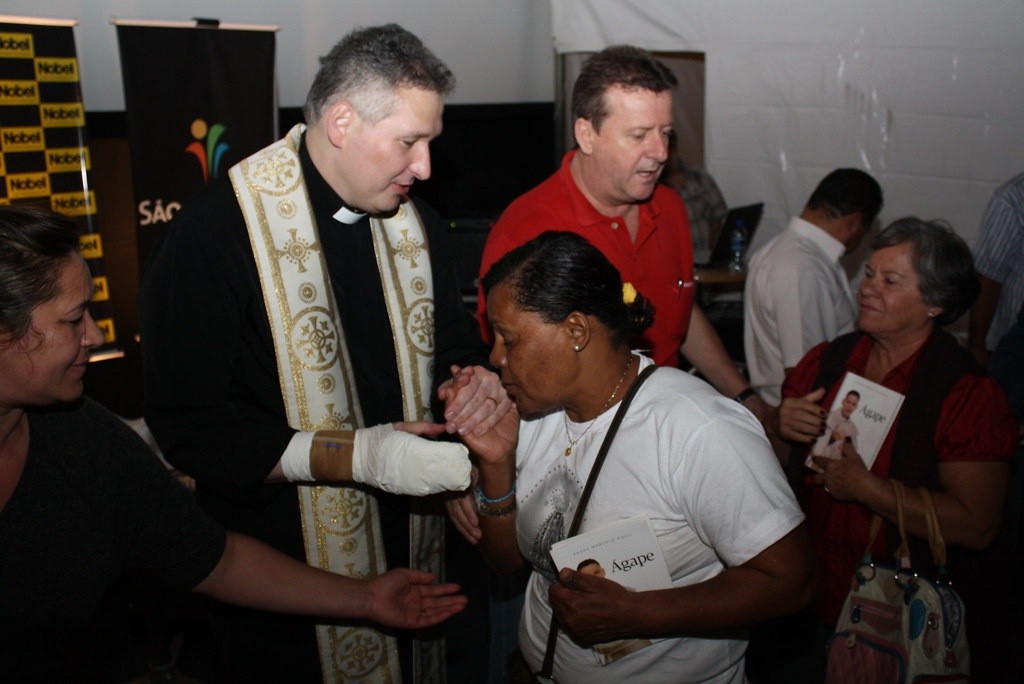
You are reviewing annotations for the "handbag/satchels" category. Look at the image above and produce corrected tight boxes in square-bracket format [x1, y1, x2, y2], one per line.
[828, 479, 971, 684]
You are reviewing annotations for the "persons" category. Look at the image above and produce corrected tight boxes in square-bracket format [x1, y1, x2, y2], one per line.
[139, 24, 513, 684]
[575, 559, 654, 665]
[0, 203, 471, 684]
[475, 44, 793, 464]
[777, 212, 1023, 684]
[447, 229, 811, 683]
[805, 390, 861, 474]
[740, 167, 884, 405]
[658, 127, 729, 267]
[967, 171, 1024, 684]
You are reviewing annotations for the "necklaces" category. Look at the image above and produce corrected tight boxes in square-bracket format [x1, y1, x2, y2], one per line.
[564, 356, 633, 456]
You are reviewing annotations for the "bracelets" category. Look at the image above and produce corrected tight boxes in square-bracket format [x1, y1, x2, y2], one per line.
[473, 485, 516, 519]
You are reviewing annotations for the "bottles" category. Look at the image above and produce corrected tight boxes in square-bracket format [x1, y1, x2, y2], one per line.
[730, 228, 745, 276]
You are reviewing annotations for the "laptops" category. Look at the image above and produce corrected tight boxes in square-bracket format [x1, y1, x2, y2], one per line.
[691, 203, 766, 270]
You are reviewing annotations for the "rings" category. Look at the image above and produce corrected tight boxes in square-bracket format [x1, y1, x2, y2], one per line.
[487, 396, 498, 407]
[823, 484, 830, 492]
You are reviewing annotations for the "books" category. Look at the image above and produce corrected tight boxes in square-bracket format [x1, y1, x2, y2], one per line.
[550, 515, 676, 665]
[804, 370, 905, 474]
